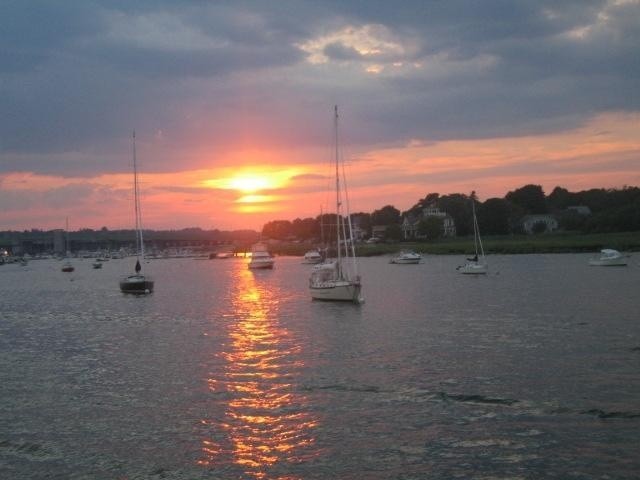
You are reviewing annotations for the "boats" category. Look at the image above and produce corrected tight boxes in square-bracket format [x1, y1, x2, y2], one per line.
[82, 248, 121, 262]
[247, 244, 273, 270]
[390, 250, 421, 264]
[62, 265, 74, 272]
[92, 263, 102, 268]
[300, 251, 323, 264]
[589, 247, 633, 267]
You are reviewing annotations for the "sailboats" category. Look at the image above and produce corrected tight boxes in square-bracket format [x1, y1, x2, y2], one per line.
[120, 128, 154, 294]
[455, 197, 490, 274]
[307, 104, 365, 304]
